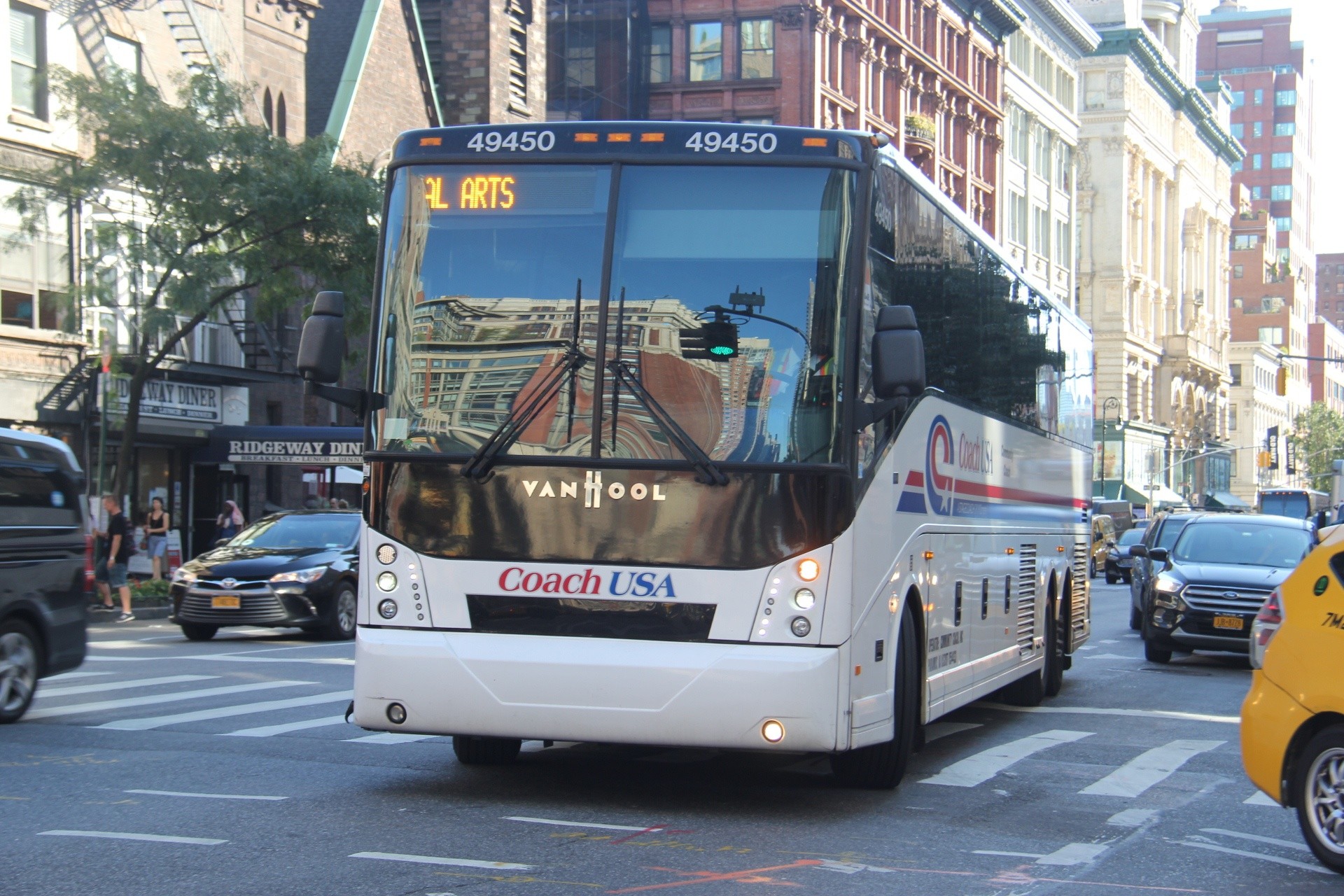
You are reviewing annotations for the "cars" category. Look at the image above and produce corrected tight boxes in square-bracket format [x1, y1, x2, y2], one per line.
[1240, 524, 1344, 874]
[1141, 512, 1319, 668]
[1089, 514, 1151, 584]
[167, 508, 362, 641]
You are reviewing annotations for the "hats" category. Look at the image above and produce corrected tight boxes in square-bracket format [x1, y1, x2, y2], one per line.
[340, 499, 348, 508]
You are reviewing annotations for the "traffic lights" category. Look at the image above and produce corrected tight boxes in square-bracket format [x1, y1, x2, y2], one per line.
[680, 322, 739, 362]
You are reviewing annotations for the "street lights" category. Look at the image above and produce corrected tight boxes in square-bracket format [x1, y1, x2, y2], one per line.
[1187, 427, 1207, 500]
[1102, 397, 1123, 495]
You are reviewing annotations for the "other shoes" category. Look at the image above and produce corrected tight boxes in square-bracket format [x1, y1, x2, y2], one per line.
[94, 603, 113, 609]
[113, 614, 136, 623]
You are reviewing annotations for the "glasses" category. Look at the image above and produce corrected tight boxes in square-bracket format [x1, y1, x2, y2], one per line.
[331, 503, 335, 504]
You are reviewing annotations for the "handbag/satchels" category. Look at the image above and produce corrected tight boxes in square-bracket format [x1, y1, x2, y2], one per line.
[208, 537, 219, 548]
[121, 521, 139, 557]
[140, 538, 147, 551]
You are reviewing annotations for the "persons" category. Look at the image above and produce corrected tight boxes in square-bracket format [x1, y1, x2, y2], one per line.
[142, 496, 170, 580]
[92, 492, 135, 623]
[215, 501, 244, 546]
[306, 498, 349, 509]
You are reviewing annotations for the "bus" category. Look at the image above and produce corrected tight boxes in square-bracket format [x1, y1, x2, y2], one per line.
[1256, 490, 1330, 520]
[298, 126, 1093, 790]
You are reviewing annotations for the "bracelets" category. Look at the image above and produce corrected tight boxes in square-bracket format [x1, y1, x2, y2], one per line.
[110, 557, 115, 560]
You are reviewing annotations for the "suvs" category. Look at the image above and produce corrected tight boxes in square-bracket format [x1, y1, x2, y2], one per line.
[0, 427, 95, 723]
[1129, 506, 1246, 643]
